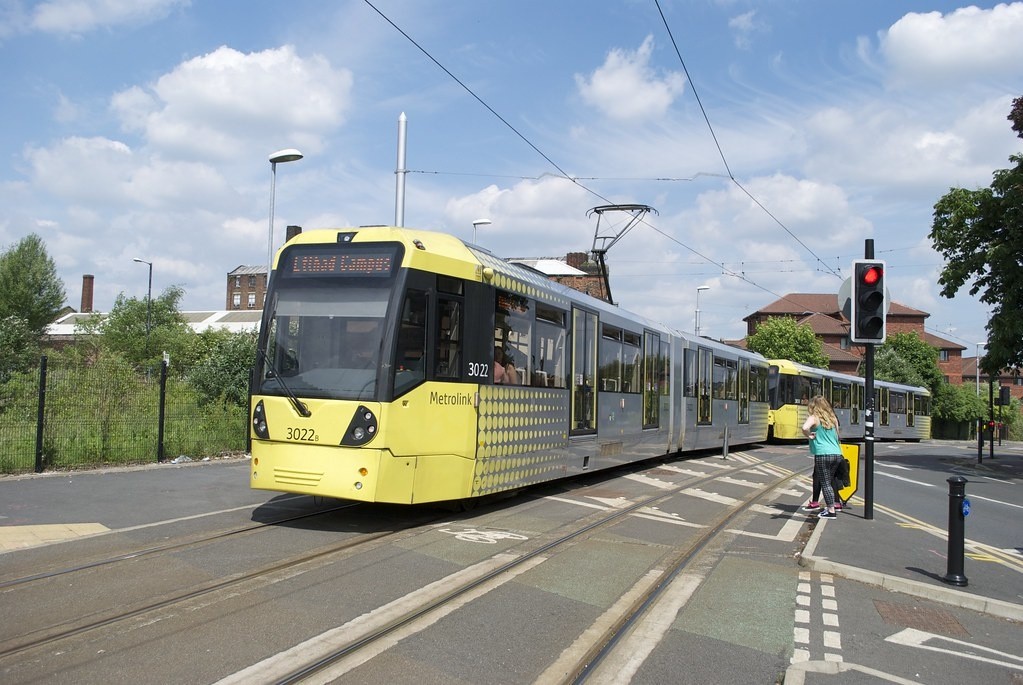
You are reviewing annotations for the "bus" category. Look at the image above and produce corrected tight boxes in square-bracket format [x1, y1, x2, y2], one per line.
[765, 323, 931, 444]
[247, 205, 770, 505]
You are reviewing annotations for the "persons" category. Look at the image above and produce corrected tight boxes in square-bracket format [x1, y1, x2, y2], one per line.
[494, 346, 517, 385]
[801, 395, 842, 520]
[801, 393, 808, 405]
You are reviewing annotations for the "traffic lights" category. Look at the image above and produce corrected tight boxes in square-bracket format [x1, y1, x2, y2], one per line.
[854, 262, 884, 341]
[987, 420, 995, 432]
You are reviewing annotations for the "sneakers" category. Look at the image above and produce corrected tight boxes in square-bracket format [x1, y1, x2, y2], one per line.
[817, 509, 837, 519]
[834, 504, 842, 511]
[801, 500, 821, 511]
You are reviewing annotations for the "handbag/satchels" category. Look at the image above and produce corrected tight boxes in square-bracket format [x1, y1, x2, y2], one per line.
[839, 457, 852, 487]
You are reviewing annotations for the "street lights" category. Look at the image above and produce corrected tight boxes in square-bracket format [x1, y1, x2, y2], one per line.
[132, 257, 153, 375]
[694, 285, 711, 335]
[261, 147, 304, 379]
[975, 342, 989, 444]
[473, 218, 492, 246]
